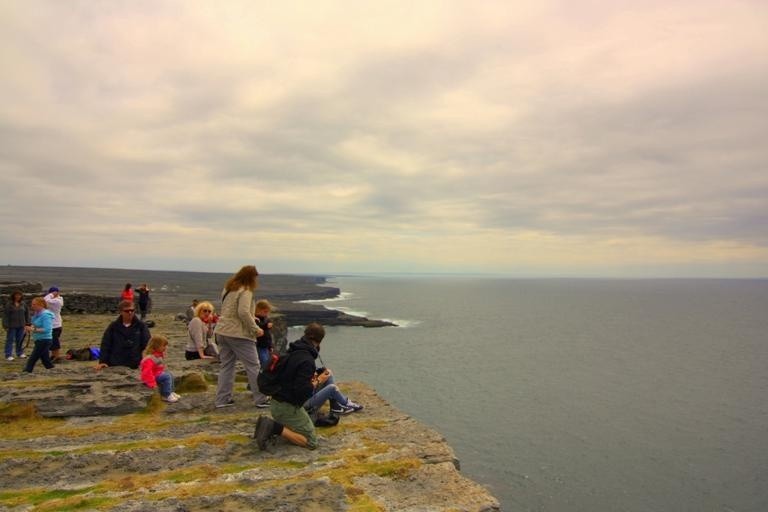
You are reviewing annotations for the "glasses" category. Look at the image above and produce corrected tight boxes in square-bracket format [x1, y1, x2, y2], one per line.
[124, 310, 134, 313]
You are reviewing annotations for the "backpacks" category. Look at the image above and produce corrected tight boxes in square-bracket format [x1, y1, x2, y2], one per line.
[257, 352, 290, 396]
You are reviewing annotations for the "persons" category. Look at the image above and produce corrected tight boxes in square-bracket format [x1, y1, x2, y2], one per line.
[252, 320, 327, 446]
[215, 265, 272, 408]
[304, 366, 361, 414]
[0, 283, 276, 402]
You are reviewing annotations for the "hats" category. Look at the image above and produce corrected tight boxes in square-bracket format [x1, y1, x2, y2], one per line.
[49, 288, 58, 291]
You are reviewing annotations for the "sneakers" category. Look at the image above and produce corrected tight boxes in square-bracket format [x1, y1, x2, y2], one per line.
[20, 354, 27, 358]
[254, 415, 274, 450]
[161, 392, 181, 403]
[5, 356, 14, 361]
[255, 398, 272, 408]
[216, 400, 235, 407]
[330, 397, 363, 415]
